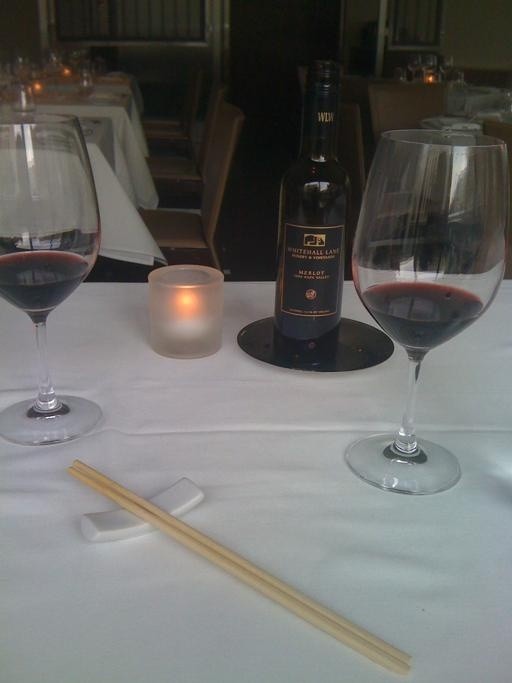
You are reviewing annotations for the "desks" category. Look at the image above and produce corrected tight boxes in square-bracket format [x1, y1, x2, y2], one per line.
[431, 79, 511, 144]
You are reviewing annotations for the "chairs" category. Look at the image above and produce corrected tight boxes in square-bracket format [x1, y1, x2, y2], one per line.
[297, 62, 368, 279]
[140, 61, 245, 272]
[367, 76, 448, 155]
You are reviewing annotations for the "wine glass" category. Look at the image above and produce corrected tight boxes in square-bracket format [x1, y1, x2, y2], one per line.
[0, 112, 114, 454]
[1, 47, 101, 116]
[344, 128, 509, 495]
[398, 49, 508, 124]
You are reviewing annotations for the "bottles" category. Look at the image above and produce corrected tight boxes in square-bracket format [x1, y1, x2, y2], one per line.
[274, 29, 353, 342]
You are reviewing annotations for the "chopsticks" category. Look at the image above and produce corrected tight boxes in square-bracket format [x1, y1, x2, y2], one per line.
[64, 458, 419, 678]
